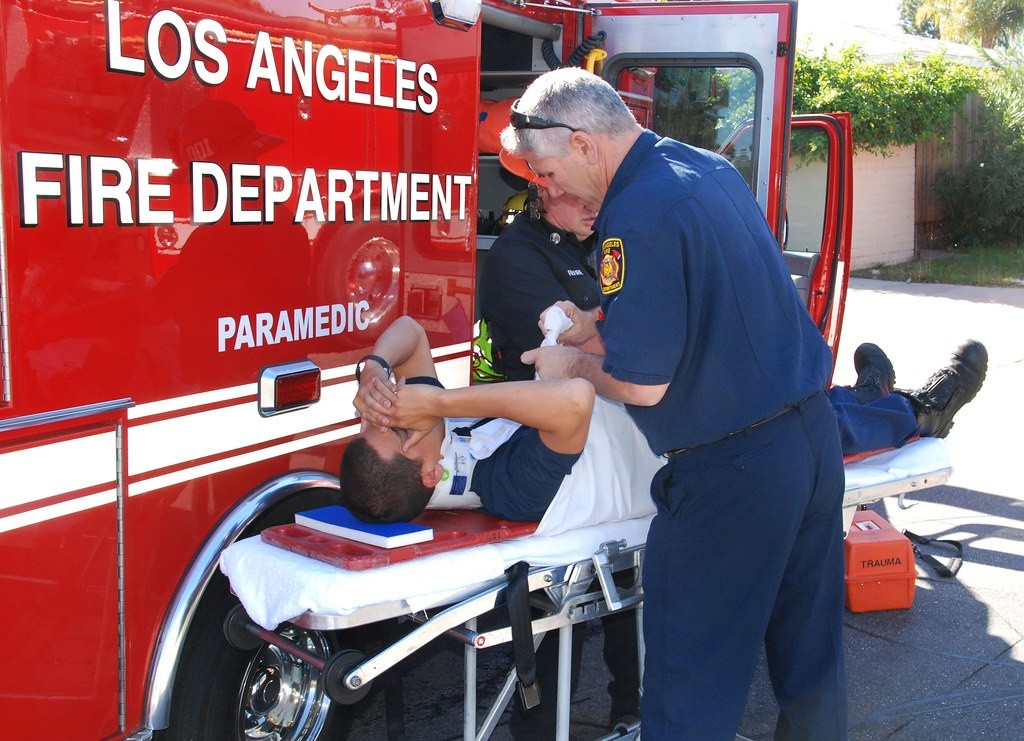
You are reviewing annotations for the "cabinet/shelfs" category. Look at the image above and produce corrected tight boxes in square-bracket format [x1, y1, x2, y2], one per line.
[477, 69, 547, 249]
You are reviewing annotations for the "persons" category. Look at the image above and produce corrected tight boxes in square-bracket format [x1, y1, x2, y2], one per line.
[503, 67, 847, 740]
[480, 177, 642, 741]
[338, 315, 988, 526]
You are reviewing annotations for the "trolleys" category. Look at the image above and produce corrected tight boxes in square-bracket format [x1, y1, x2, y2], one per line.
[217, 436, 958, 741]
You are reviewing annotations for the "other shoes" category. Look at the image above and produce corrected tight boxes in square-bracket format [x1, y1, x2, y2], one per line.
[895, 339, 988, 438]
[854, 342, 896, 396]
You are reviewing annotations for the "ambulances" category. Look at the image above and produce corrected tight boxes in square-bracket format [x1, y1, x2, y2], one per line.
[0, 0, 853, 739]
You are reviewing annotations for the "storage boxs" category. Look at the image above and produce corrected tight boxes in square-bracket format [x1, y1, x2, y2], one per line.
[845, 510, 920, 611]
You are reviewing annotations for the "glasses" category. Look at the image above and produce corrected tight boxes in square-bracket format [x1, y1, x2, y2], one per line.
[509, 98, 577, 132]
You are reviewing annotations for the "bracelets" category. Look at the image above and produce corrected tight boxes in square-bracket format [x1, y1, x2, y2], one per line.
[355, 355, 392, 385]
